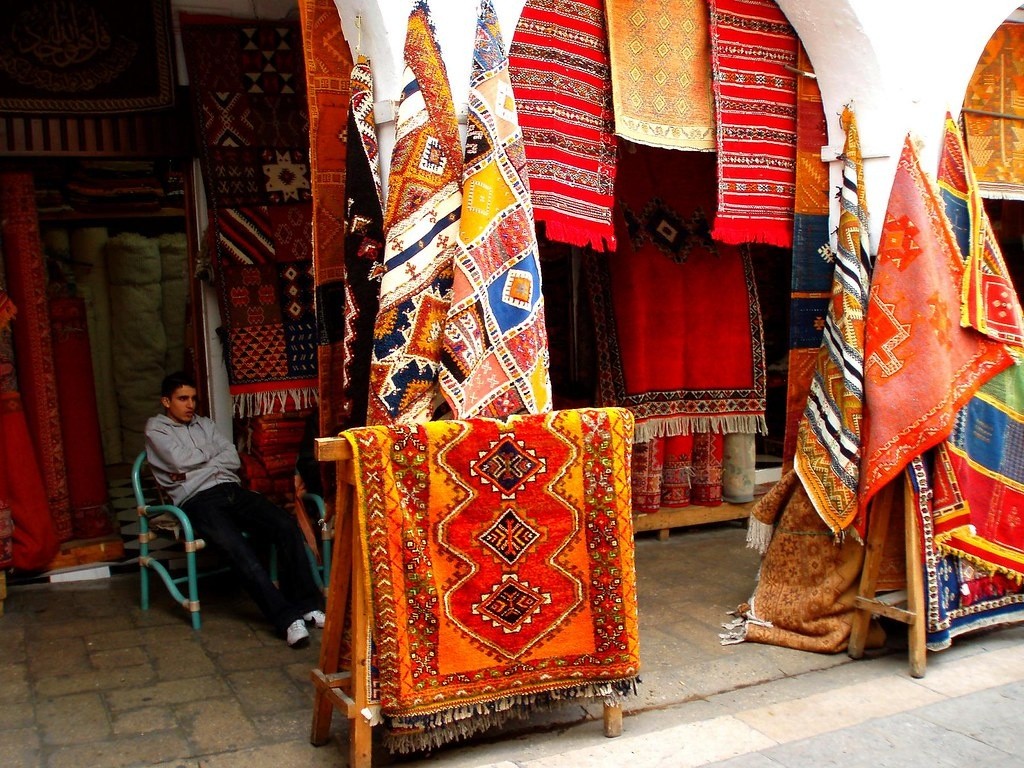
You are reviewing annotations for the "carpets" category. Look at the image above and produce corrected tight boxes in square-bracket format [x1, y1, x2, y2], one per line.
[1, 0, 1023, 755]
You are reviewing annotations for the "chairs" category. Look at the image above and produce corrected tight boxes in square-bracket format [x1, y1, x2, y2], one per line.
[293, 451, 331, 608]
[132, 449, 279, 630]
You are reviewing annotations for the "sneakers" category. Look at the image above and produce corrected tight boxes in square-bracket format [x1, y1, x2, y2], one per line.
[286, 619, 309, 646]
[303, 609, 325, 628]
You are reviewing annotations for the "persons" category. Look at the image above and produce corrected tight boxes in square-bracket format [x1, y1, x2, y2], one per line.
[144, 372, 327, 647]
